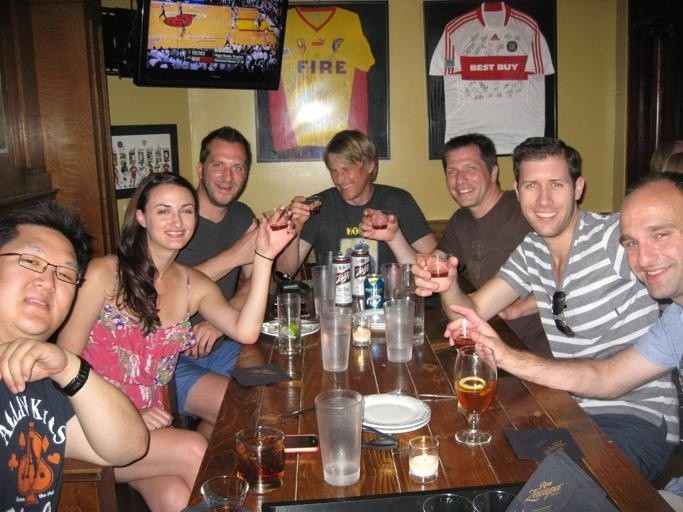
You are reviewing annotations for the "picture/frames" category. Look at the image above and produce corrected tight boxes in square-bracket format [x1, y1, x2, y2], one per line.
[107, 122, 180, 199]
[252, 1, 392, 164]
[422, 1, 558, 160]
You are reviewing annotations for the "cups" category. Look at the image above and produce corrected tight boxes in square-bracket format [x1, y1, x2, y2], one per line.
[314, 389, 363, 486]
[368, 209, 389, 229]
[304, 195, 321, 215]
[473, 491, 525, 512]
[199, 474, 249, 512]
[273, 242, 452, 370]
[406, 434, 439, 486]
[262, 208, 289, 231]
[421, 493, 476, 512]
[235, 426, 285, 494]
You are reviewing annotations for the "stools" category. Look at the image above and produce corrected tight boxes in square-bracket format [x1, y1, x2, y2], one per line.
[51, 450, 121, 512]
[161, 369, 181, 430]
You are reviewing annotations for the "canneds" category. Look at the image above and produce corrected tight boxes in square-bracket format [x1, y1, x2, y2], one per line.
[351, 250, 371, 298]
[332, 256, 353, 307]
[364, 274, 385, 310]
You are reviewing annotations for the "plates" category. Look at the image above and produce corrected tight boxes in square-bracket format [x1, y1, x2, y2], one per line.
[359, 393, 432, 435]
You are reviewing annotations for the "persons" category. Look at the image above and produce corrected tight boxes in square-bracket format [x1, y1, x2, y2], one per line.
[114, 138, 170, 188]
[411, 137, 683, 484]
[358, 133, 556, 362]
[147, 0, 282, 75]
[273, 129, 438, 283]
[57, 172, 298, 511]
[0, 196, 151, 512]
[167, 127, 260, 440]
[445, 169, 683, 511]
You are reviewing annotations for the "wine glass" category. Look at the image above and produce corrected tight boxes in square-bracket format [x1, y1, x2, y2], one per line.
[452, 345, 495, 446]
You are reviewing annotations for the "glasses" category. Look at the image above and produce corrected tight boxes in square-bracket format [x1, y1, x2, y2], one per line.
[0, 248, 85, 287]
[552, 290, 578, 339]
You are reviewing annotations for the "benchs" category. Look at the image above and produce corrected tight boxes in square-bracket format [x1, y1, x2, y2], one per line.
[267, 217, 454, 294]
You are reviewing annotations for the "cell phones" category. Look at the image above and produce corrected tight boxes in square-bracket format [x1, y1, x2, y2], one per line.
[269, 309, 312, 320]
[283, 434, 320, 453]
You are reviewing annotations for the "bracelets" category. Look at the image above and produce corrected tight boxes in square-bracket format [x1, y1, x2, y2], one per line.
[53, 355, 90, 397]
[255, 251, 273, 261]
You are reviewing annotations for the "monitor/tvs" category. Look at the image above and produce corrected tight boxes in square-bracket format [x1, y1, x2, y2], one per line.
[133, 0, 288, 90]
